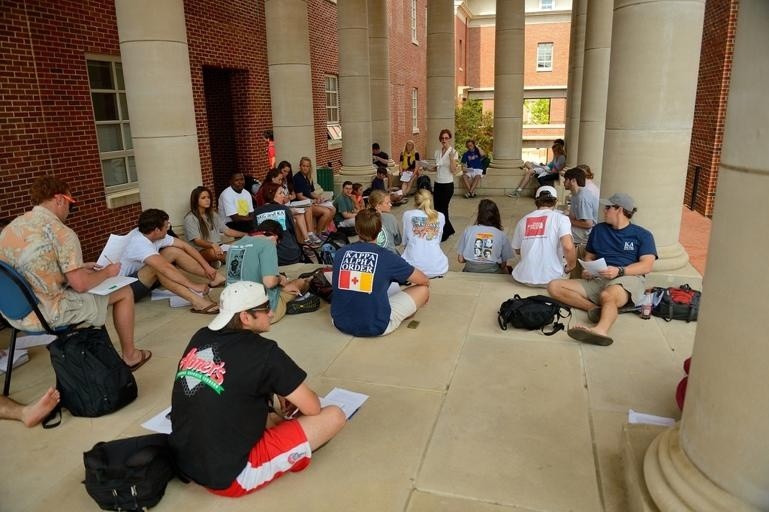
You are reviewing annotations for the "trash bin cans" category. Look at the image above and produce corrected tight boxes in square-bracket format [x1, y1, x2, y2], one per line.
[317, 166, 334, 191]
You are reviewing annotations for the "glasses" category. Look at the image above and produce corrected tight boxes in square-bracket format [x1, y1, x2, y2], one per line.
[248, 304, 272, 314]
[53, 193, 81, 213]
[440, 137, 450, 140]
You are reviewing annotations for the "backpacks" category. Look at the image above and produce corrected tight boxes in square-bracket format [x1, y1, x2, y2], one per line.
[495, 291, 572, 337]
[320, 230, 350, 266]
[296, 266, 334, 304]
[42, 325, 140, 418]
[81, 432, 190, 512]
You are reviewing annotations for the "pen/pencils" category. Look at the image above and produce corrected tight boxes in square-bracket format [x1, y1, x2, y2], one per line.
[287, 278, 303, 297]
[104, 255, 113, 264]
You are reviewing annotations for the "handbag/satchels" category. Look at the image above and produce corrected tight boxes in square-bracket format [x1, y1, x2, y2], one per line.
[651, 283, 701, 323]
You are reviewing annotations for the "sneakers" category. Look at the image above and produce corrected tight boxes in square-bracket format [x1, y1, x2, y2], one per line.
[307, 231, 322, 244]
[285, 291, 321, 314]
[464, 192, 472, 198]
[472, 192, 475, 198]
[304, 239, 320, 249]
[507, 191, 521, 198]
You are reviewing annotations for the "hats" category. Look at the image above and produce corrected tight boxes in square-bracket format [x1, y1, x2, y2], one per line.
[534, 185, 558, 199]
[248, 219, 284, 239]
[206, 279, 270, 332]
[597, 191, 635, 214]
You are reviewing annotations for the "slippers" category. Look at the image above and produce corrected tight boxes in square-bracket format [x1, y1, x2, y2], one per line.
[123, 348, 153, 372]
[587, 306, 602, 323]
[566, 326, 615, 348]
[189, 302, 220, 314]
[207, 279, 226, 288]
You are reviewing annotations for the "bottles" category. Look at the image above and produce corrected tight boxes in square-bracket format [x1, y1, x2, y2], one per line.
[640, 287, 652, 320]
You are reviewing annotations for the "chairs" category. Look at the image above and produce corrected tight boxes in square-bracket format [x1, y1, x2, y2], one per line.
[0, 259, 95, 401]
